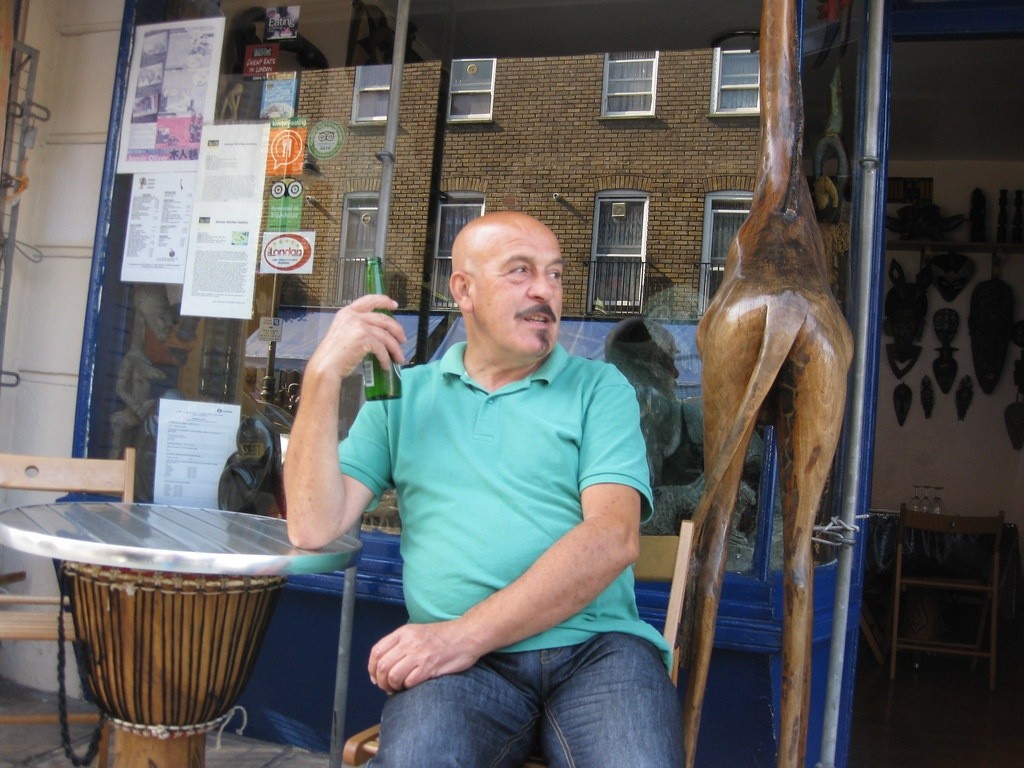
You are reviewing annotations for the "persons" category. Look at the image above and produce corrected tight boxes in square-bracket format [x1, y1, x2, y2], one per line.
[283, 210, 688, 768]
[600, 316, 763, 536]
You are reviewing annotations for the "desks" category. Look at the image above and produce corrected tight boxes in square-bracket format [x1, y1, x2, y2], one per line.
[0, 499, 364, 768]
[866, 511, 1018, 658]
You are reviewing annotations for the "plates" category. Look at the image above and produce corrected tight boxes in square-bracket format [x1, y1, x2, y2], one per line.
[870, 508, 900, 513]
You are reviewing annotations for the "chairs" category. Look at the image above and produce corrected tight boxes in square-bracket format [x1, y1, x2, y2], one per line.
[1, 445, 137, 768]
[891, 502, 1005, 690]
[342, 518, 696, 767]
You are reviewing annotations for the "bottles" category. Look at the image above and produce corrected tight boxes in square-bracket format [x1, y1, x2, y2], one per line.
[362, 257, 402, 401]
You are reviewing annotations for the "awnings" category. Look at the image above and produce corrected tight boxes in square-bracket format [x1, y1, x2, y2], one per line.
[241, 306, 701, 399]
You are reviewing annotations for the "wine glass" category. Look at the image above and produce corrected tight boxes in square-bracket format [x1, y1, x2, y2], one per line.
[931, 487, 945, 515]
[909, 486, 922, 512]
[920, 486, 931, 514]
